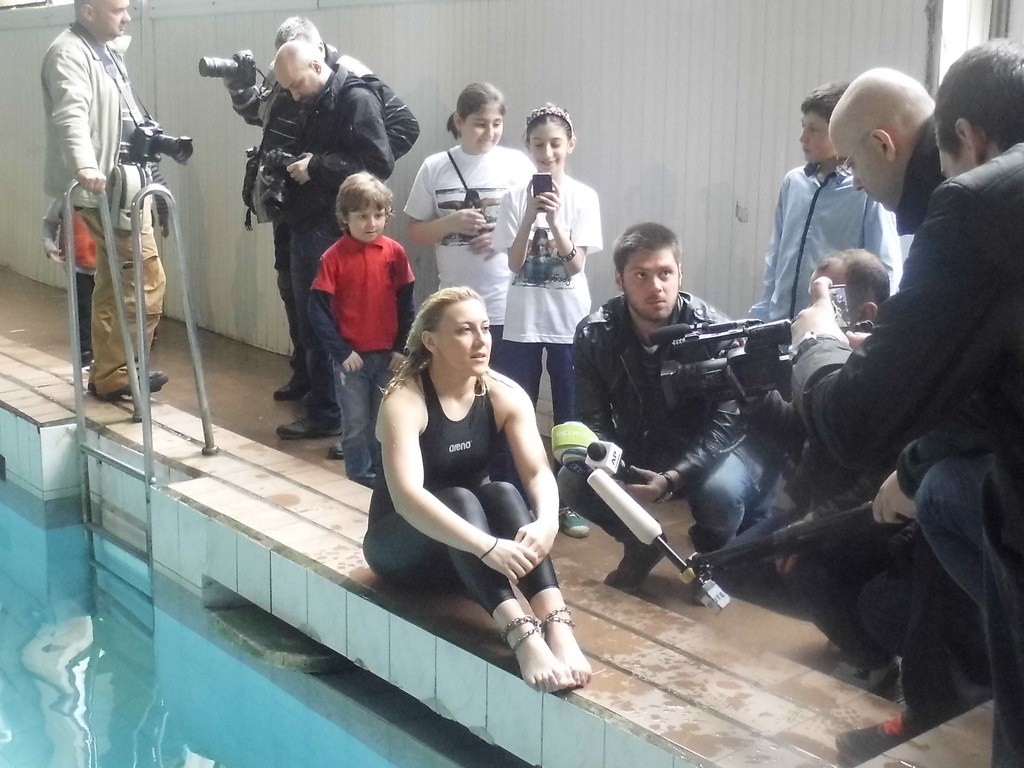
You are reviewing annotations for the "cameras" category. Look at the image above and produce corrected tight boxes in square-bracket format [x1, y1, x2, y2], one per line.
[130, 120, 193, 165]
[265, 149, 302, 203]
[199, 50, 256, 89]
[827, 283, 852, 334]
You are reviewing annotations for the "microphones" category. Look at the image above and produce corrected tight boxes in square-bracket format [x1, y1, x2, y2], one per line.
[586, 468, 696, 584]
[648, 322, 708, 346]
[584, 441, 647, 487]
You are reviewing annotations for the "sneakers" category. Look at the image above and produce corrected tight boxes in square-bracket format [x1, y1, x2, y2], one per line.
[277, 416, 342, 439]
[828, 640, 900, 696]
[835, 707, 948, 765]
[559, 506, 590, 539]
[328, 440, 344, 459]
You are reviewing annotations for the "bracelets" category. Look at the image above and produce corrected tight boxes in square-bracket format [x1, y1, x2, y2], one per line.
[480, 538, 498, 560]
[556, 243, 577, 261]
[786, 332, 840, 367]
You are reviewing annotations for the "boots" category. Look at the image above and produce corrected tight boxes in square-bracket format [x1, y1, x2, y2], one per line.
[604, 519, 669, 593]
[679, 526, 736, 602]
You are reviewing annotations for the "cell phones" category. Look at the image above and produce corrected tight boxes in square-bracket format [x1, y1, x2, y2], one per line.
[532, 173, 553, 199]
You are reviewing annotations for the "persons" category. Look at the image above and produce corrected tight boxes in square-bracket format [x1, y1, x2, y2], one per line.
[363, 285, 591, 694]
[402, 80, 539, 374]
[42, 0, 171, 401]
[493, 103, 604, 471]
[556, 222, 772, 605]
[224, 17, 419, 487]
[747, 40, 1024, 767]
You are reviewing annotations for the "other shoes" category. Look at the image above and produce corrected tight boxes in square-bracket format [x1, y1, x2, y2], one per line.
[81, 351, 92, 364]
[88, 372, 169, 400]
[273, 377, 312, 408]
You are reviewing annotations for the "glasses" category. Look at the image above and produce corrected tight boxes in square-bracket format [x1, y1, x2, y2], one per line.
[834, 130, 873, 177]
